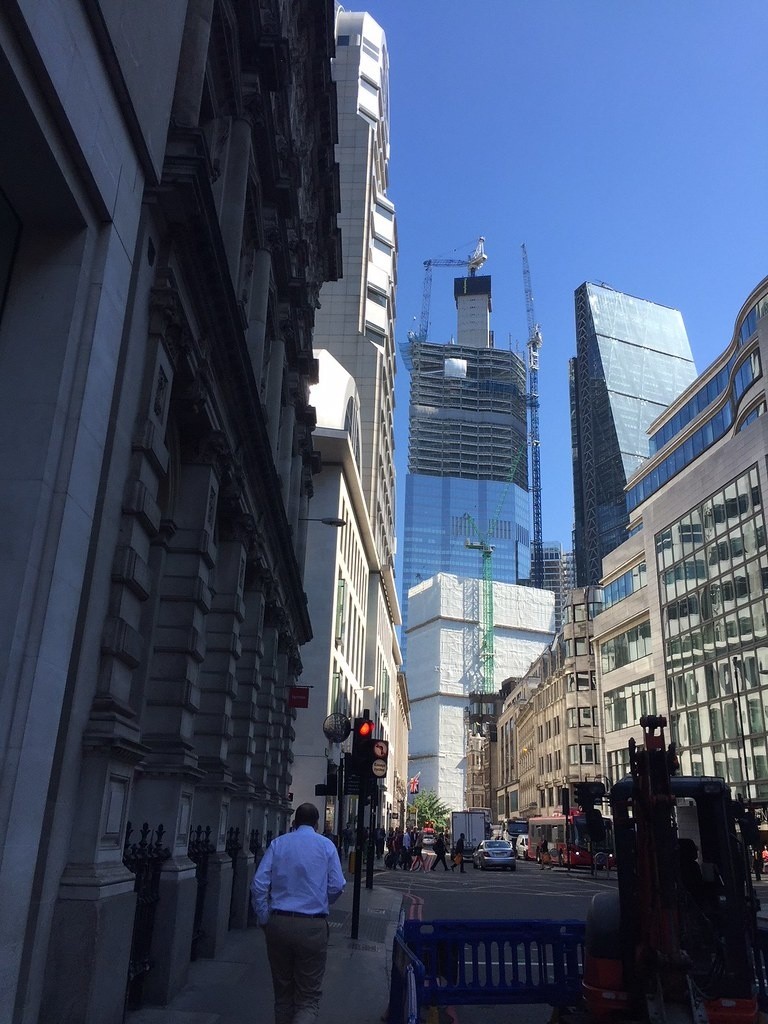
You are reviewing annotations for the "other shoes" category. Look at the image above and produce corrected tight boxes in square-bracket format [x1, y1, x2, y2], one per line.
[431, 867, 436, 871]
[539, 868, 544, 870]
[461, 870, 467, 873]
[445, 868, 450, 871]
[451, 866, 454, 871]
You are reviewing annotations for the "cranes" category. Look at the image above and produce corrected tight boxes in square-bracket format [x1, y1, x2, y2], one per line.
[459, 439, 534, 690]
[519, 242, 545, 587]
[418, 236, 487, 344]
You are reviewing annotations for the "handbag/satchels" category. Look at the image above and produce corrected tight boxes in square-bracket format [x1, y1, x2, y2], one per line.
[414, 847, 420, 855]
[433, 844, 441, 854]
[454, 855, 462, 865]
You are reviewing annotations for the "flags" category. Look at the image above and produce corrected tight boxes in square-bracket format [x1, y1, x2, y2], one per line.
[410, 775, 419, 794]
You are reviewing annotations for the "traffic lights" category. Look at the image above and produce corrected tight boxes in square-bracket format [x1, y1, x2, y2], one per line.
[573, 783, 583, 805]
[351, 719, 374, 772]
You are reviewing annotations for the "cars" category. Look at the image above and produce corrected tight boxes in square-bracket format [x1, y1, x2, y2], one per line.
[422, 835, 436, 845]
[472, 840, 516, 871]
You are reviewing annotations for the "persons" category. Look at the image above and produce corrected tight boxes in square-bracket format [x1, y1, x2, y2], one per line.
[430, 833, 451, 871]
[250, 803, 348, 1024]
[539, 835, 553, 870]
[388, 825, 428, 874]
[323, 823, 385, 863]
[450, 833, 467, 873]
[753, 858, 763, 881]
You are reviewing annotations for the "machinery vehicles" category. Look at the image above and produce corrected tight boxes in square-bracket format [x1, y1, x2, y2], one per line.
[551, 715, 768, 1024]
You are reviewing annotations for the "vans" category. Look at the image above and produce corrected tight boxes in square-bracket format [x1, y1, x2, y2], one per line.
[516, 834, 529, 860]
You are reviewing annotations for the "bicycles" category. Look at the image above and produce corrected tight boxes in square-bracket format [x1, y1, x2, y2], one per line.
[384, 849, 420, 871]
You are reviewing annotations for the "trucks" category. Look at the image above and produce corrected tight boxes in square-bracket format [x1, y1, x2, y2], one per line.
[492, 825, 503, 840]
[497, 817, 528, 850]
[451, 811, 486, 861]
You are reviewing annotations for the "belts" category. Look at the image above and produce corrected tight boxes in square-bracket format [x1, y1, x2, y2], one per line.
[271, 910, 327, 918]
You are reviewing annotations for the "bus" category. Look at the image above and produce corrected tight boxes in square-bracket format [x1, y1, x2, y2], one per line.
[528, 816, 615, 870]
[467, 807, 492, 840]
